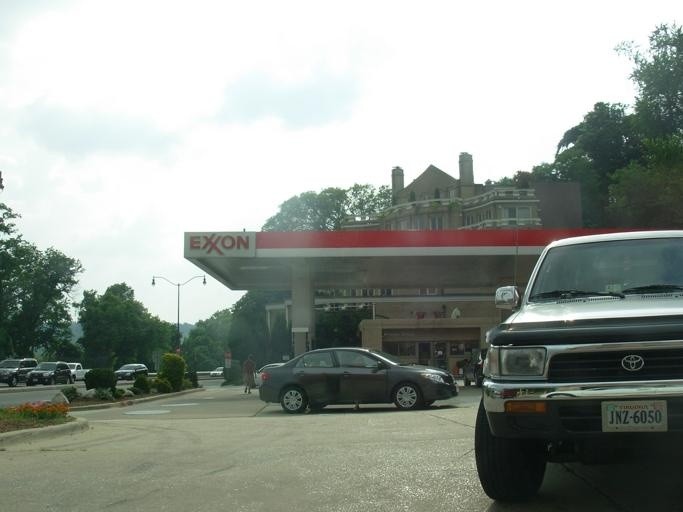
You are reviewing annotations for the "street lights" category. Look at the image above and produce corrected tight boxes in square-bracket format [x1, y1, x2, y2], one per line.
[151, 274, 206, 355]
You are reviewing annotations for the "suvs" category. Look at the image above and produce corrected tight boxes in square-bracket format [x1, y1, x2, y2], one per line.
[0, 358, 38, 387]
[24, 361, 71, 386]
[474, 229, 682, 503]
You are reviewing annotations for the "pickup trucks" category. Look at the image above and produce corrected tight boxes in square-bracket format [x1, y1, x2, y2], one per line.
[66, 362, 92, 384]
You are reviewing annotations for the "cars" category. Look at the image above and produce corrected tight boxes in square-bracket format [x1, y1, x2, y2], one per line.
[251, 362, 285, 388]
[462, 348, 487, 387]
[209, 366, 224, 377]
[113, 363, 148, 380]
[259, 347, 459, 414]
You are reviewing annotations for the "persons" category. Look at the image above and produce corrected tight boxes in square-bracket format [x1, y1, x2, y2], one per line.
[241, 353, 256, 394]
